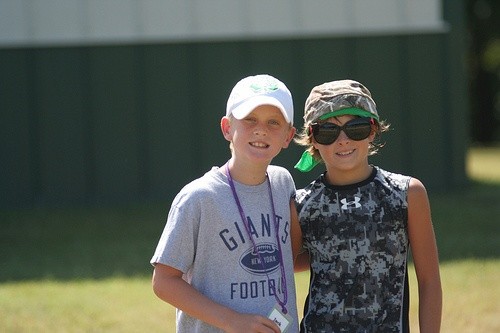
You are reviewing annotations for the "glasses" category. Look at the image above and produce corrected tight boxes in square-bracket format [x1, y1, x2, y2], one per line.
[310, 117, 371, 145]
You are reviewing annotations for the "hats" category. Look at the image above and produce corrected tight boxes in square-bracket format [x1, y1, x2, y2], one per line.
[226, 74, 294, 127]
[294, 80, 379, 173]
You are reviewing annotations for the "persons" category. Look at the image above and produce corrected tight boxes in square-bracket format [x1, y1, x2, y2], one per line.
[293, 80, 443, 332]
[148, 75, 308, 332]
